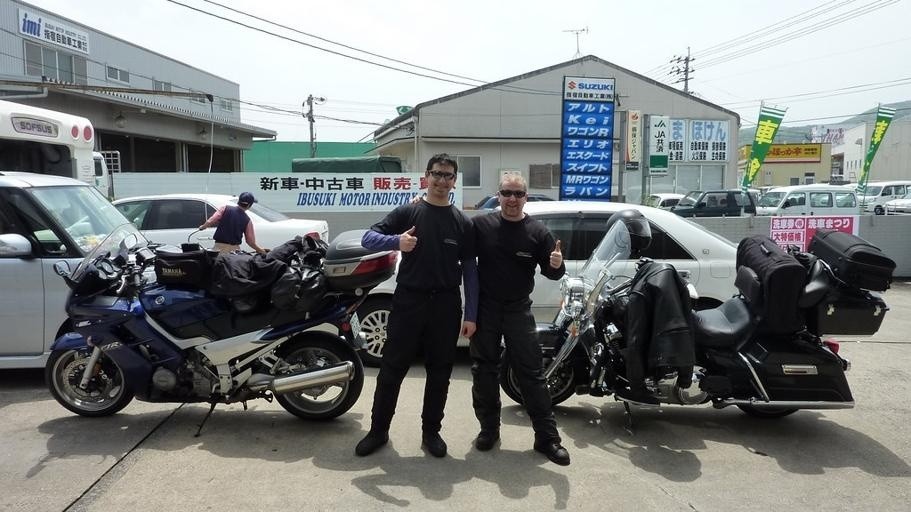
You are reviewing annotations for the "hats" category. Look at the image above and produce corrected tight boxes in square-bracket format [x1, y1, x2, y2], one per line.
[238, 192, 257, 207]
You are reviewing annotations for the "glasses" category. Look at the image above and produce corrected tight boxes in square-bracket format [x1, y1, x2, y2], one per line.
[430, 170, 456, 180]
[499, 190, 526, 198]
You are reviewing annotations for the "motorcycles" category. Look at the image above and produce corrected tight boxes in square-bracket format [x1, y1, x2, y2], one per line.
[493, 219, 895, 440]
[43, 221, 396, 438]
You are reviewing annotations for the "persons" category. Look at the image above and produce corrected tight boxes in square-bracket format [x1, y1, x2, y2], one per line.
[354, 152, 480, 462]
[194, 188, 266, 256]
[408, 193, 423, 204]
[465, 170, 571, 467]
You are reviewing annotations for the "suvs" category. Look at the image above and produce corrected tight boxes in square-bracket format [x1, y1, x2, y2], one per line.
[660, 189, 756, 216]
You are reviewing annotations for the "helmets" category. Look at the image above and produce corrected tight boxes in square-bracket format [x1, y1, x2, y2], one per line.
[605, 209, 652, 252]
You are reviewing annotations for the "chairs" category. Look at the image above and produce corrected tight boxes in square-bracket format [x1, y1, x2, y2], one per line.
[793, 250, 830, 310]
[692, 263, 764, 344]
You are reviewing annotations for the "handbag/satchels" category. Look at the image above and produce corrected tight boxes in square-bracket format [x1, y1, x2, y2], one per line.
[735, 234, 804, 334]
[153, 245, 212, 290]
[271, 251, 327, 312]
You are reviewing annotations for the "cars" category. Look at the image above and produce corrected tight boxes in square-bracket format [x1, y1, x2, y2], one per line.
[62, 195, 327, 256]
[351, 199, 748, 368]
[648, 193, 695, 210]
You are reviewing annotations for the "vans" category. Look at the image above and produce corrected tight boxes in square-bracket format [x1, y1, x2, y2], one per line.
[750, 180, 911, 215]
[0, 170, 184, 369]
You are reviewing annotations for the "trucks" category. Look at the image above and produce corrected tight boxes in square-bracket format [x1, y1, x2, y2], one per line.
[0, 99, 112, 202]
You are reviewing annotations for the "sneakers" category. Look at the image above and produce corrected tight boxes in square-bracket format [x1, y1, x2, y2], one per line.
[356, 428, 388, 457]
[422, 433, 446, 456]
[477, 430, 499, 450]
[534, 440, 570, 465]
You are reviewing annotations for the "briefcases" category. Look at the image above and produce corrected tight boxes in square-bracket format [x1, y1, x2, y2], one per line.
[808, 228, 895, 291]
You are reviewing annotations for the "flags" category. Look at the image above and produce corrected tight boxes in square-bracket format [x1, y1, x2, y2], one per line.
[856, 106, 898, 193]
[741, 104, 785, 186]
